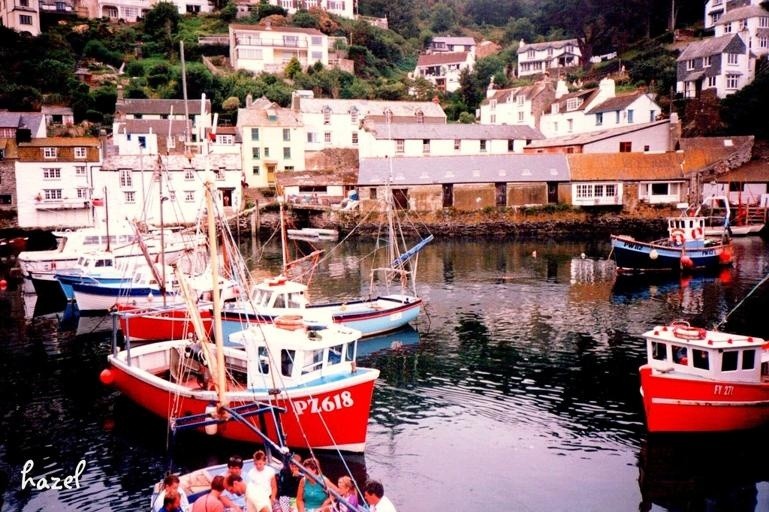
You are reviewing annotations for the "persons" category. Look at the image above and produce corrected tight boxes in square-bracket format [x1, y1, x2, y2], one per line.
[152, 447, 399, 512]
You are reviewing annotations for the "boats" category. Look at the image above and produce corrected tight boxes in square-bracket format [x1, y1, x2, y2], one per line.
[639, 325, 769, 433]
[610, 195, 736, 277]
